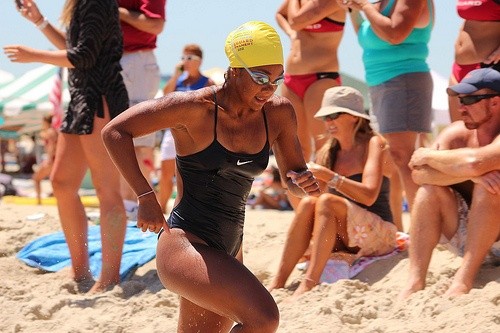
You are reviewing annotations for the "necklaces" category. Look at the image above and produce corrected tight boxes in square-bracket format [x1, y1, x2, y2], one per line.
[349, 0, 386, 13]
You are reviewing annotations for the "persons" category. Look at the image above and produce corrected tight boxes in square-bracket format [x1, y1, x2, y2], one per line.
[400, 67, 500, 300]
[101, 20, 320, 333]
[0, 135, 39, 176]
[93, 0, 166, 226]
[32, 115, 59, 205]
[337, 0, 435, 232]
[449, 0, 500, 122]
[275, 0, 349, 165]
[266, 87, 398, 298]
[250, 165, 294, 211]
[4, 0, 130, 296]
[156, 43, 216, 214]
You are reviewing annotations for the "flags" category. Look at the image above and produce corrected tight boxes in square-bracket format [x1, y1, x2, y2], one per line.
[48, 66, 64, 129]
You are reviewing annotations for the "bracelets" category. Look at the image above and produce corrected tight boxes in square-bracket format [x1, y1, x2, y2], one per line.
[136, 190, 155, 199]
[36, 18, 49, 31]
[34, 15, 42, 23]
[336, 176, 345, 191]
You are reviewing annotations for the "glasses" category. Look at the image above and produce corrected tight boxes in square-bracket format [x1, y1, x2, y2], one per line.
[181, 56, 198, 60]
[459, 94, 500, 105]
[231, 37, 284, 86]
[322, 112, 348, 120]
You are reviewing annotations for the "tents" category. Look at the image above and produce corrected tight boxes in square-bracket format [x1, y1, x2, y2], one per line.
[0, 63, 175, 139]
[200, 66, 228, 86]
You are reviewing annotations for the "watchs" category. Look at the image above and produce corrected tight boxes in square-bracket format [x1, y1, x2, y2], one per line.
[327, 173, 339, 188]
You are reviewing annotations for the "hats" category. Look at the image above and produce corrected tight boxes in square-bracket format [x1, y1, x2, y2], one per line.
[313, 86, 371, 120]
[446, 67, 500, 96]
[225, 21, 284, 68]
